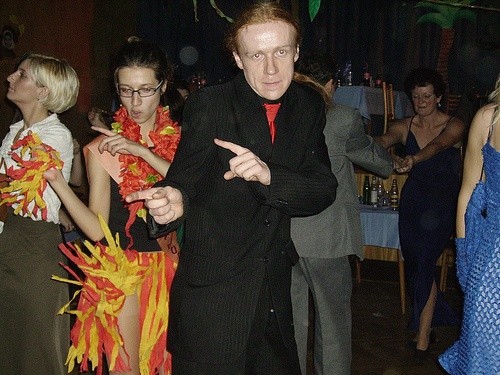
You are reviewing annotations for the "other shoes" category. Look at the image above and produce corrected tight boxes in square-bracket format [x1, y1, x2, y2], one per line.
[409, 326, 435, 360]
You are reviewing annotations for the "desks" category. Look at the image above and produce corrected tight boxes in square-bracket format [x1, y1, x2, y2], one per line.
[354, 171, 409, 315]
[333, 85, 416, 135]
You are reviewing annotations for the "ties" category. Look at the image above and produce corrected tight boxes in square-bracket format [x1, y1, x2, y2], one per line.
[263, 102, 282, 145]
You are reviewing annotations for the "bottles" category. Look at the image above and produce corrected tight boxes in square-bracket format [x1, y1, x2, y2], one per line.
[363, 176, 371, 205]
[376, 75, 381, 88]
[363, 64, 371, 86]
[371, 176, 378, 205]
[377, 177, 384, 206]
[391, 179, 399, 210]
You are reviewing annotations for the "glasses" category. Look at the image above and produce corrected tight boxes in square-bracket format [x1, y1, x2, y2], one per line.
[116, 79, 163, 98]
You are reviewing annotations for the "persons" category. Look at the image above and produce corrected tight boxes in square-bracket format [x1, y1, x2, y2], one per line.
[0, 42, 500, 375]
[124, 3, 338, 375]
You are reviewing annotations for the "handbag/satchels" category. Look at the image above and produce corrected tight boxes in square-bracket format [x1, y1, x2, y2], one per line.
[0, 157, 11, 223]
